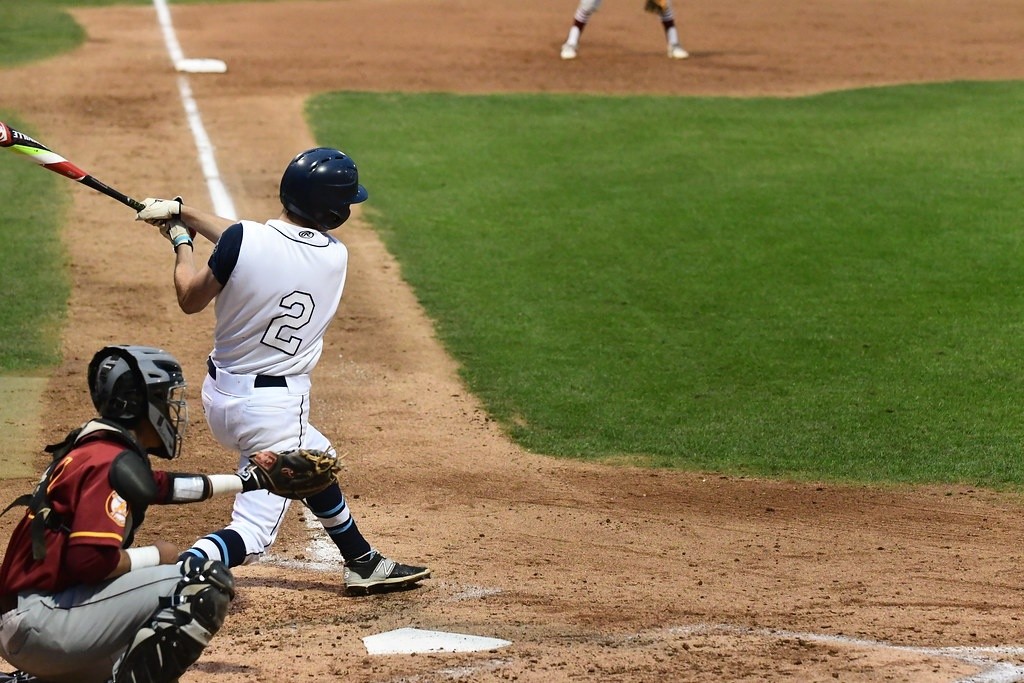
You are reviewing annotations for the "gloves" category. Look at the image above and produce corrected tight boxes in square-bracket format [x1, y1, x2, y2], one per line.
[135, 197, 182, 228]
[159, 196, 197, 253]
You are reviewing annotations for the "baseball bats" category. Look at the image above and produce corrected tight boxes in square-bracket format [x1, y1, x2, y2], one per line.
[0, 121, 145, 211]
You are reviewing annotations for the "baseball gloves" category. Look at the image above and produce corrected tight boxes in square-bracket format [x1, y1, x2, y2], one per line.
[249, 449, 342, 500]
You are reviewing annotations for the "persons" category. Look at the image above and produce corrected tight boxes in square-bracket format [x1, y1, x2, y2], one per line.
[135, 147, 431, 594]
[560, 0, 689, 59]
[0, 346, 345, 683]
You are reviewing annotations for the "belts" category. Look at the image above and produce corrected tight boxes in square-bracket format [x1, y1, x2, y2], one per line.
[0, 595, 18, 614]
[208, 356, 287, 387]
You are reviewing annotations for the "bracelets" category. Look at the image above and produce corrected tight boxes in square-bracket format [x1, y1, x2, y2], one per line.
[171, 234, 194, 253]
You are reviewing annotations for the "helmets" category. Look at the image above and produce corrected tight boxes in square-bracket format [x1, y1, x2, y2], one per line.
[280, 148, 368, 229]
[88, 345, 187, 460]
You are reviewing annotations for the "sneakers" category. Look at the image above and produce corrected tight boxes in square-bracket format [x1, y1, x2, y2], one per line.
[344, 551, 430, 596]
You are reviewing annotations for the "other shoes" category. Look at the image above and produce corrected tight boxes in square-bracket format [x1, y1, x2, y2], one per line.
[667, 45, 689, 58]
[561, 42, 577, 59]
